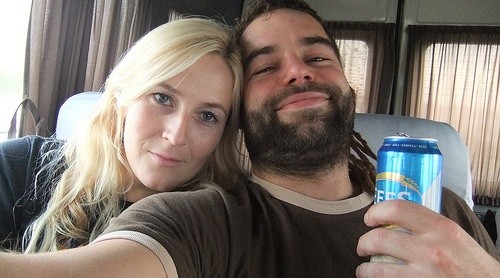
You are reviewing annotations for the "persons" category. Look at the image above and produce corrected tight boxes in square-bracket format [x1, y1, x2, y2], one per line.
[0, 1, 500, 278]
[0, 16, 247, 257]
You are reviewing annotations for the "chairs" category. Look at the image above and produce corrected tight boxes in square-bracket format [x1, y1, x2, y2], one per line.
[242, 112, 472, 213]
[54, 93, 241, 212]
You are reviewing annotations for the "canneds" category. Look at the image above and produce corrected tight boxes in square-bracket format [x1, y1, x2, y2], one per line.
[373, 132, 442, 232]
